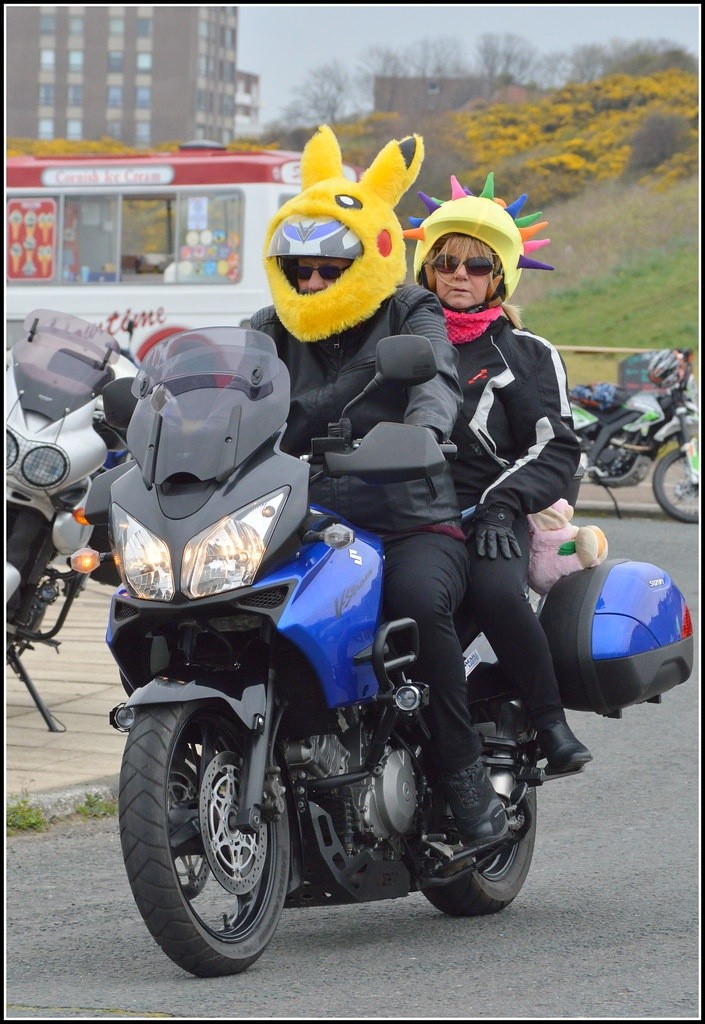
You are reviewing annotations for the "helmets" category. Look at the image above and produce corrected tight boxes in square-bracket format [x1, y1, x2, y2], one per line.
[266, 213, 364, 260]
[649, 348, 684, 388]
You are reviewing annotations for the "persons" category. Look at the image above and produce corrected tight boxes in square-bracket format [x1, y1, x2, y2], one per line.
[401, 172, 593, 777]
[181, 124, 510, 846]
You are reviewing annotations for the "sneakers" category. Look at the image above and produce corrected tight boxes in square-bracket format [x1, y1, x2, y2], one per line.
[442, 757, 508, 847]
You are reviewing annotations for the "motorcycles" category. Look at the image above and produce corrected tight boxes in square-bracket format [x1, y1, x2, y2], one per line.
[4, 308, 233, 732]
[567, 348, 699, 525]
[69, 325, 693, 981]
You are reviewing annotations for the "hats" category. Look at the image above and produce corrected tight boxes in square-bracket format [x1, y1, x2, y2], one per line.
[403, 171, 554, 312]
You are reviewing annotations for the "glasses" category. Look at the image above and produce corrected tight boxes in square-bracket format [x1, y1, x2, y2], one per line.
[434, 254, 495, 276]
[288, 264, 352, 280]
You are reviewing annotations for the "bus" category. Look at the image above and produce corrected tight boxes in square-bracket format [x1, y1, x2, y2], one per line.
[6, 139, 358, 381]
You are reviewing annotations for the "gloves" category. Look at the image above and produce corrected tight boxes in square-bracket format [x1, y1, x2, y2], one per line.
[463, 507, 523, 559]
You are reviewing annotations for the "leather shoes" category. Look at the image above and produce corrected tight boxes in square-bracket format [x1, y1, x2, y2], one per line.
[537, 720, 591, 772]
